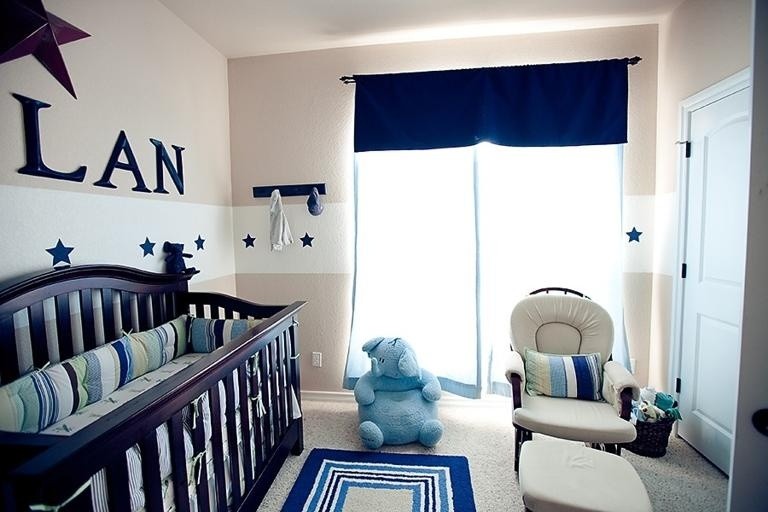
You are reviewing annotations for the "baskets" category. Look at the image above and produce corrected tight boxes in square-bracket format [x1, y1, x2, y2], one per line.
[621, 408, 675, 459]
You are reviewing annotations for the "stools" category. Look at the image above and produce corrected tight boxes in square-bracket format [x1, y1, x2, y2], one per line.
[519, 440, 653, 512]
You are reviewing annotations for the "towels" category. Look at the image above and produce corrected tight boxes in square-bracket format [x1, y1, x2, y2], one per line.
[269, 188, 294, 252]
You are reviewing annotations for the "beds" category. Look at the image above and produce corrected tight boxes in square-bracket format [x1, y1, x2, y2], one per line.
[0, 264, 308, 512]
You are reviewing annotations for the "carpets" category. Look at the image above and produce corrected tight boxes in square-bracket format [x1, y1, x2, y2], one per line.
[279, 447, 477, 512]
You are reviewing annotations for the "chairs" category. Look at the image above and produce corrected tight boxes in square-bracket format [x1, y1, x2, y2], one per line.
[503, 287, 641, 474]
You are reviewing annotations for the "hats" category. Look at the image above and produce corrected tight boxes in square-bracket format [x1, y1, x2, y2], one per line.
[306, 188, 323, 216]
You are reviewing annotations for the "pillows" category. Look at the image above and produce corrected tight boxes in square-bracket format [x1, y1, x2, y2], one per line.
[0, 314, 262, 433]
[523, 348, 605, 403]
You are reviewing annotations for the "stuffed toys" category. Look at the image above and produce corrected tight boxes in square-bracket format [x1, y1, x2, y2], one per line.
[352, 336, 445, 447]
